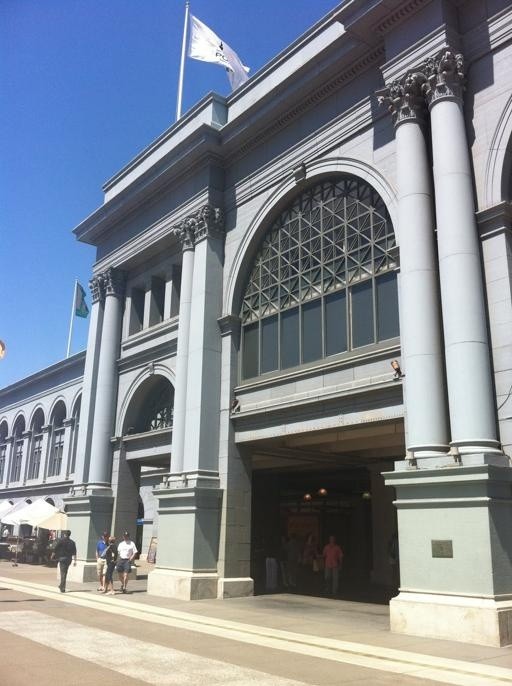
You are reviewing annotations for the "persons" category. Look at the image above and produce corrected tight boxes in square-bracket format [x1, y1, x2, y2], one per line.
[100, 537, 119, 596]
[51, 530, 78, 593]
[96, 531, 110, 591]
[251, 532, 344, 595]
[117, 531, 139, 594]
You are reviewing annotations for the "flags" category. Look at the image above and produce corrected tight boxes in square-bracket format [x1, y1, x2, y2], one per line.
[75, 282, 90, 319]
[187, 10, 251, 93]
[0, 339, 7, 359]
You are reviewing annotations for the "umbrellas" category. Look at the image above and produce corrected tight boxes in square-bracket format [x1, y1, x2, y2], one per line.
[0, 499, 70, 540]
